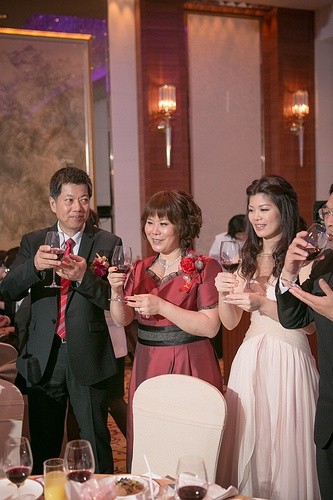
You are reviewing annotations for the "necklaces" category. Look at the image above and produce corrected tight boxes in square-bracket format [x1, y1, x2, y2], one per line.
[157, 253, 181, 269]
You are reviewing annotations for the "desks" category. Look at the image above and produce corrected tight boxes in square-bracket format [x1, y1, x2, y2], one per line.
[25, 474, 268, 500]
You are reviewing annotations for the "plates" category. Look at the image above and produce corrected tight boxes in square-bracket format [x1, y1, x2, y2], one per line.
[103, 475, 148, 498]
[97, 475, 161, 500]
[0, 478, 44, 500]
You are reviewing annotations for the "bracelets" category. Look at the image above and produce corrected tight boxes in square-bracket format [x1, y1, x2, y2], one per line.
[108, 297, 125, 302]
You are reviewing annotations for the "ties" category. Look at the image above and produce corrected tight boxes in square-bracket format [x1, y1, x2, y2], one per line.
[57, 240, 75, 339]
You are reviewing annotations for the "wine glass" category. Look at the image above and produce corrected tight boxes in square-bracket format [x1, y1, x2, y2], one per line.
[3, 436, 33, 499]
[43, 231, 66, 288]
[220, 241, 243, 303]
[108, 245, 133, 303]
[280, 222, 328, 291]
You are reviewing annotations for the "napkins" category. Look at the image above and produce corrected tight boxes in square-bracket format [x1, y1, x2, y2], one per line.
[208, 484, 238, 500]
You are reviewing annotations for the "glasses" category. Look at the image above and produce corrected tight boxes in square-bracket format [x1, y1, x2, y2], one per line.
[318, 207, 333, 219]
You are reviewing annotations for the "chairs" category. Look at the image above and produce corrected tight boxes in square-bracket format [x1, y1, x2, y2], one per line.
[0, 343, 25, 477]
[132, 373, 228, 485]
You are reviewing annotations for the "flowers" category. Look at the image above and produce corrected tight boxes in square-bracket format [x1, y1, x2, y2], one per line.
[179, 253, 204, 281]
[90, 253, 110, 278]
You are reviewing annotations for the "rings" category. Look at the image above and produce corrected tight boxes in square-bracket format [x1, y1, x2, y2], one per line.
[138, 307, 141, 312]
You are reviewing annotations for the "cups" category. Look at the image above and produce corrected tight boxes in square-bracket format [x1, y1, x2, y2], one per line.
[42, 458, 69, 500]
[63, 440, 95, 483]
[175, 455, 209, 500]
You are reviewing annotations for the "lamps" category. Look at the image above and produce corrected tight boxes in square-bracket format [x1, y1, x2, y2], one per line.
[290, 90, 309, 168]
[158, 84, 176, 169]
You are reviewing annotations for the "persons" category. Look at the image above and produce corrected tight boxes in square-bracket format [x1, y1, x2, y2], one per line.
[0, 165, 333, 500]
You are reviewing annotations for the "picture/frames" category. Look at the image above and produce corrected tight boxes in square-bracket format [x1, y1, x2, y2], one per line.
[0, 27, 99, 251]
